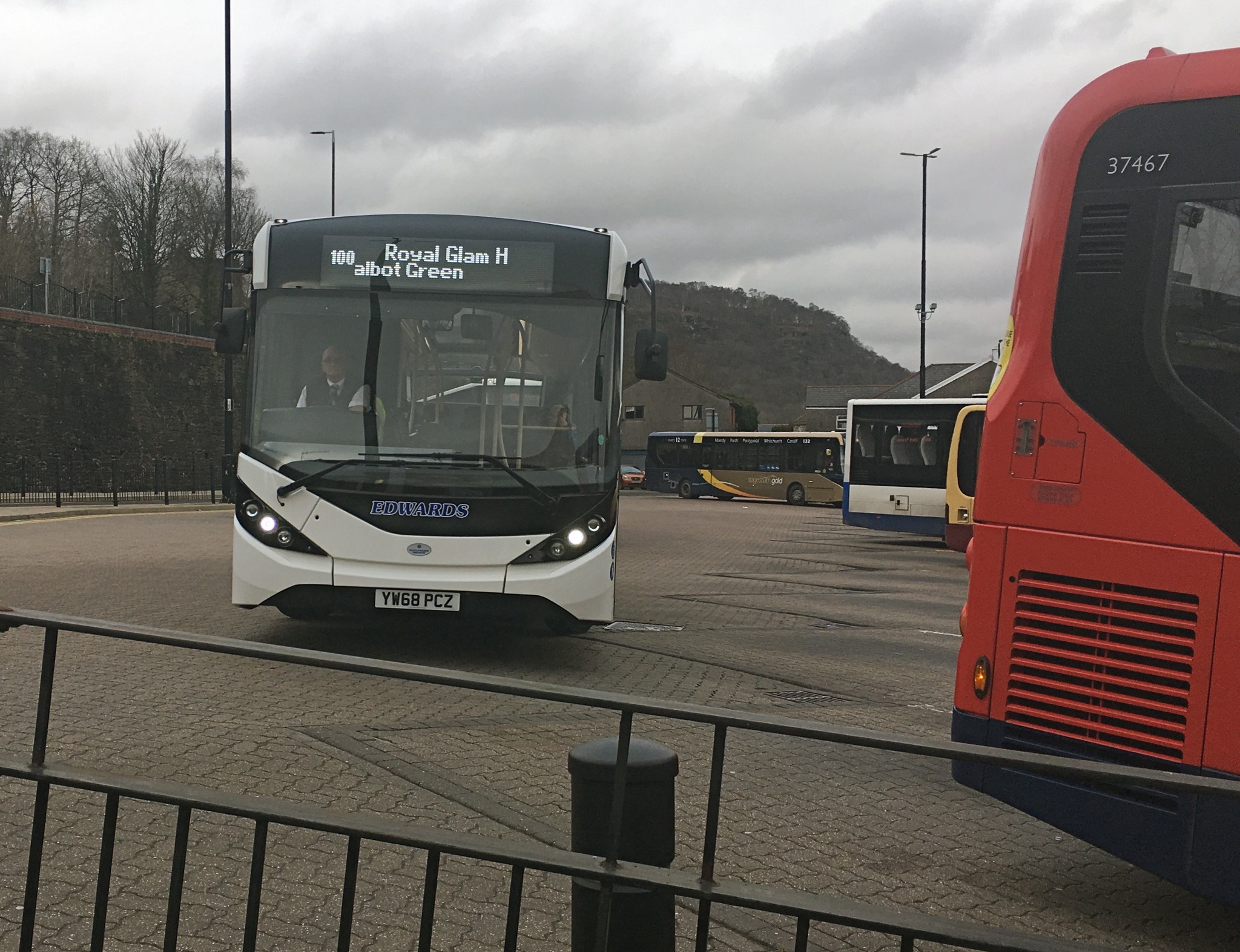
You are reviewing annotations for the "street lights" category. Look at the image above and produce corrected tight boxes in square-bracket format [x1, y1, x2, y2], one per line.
[901, 148, 942, 399]
[310, 130, 335, 216]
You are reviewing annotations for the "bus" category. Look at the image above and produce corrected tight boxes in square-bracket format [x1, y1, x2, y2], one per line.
[644, 430, 845, 507]
[950, 39, 1239, 899]
[212, 213, 670, 640]
[942, 404, 986, 553]
[842, 393, 988, 537]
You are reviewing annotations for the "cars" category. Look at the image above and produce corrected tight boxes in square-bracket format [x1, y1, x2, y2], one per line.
[619, 464, 645, 490]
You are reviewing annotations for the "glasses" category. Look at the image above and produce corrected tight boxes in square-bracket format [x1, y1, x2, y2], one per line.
[322, 356, 343, 363]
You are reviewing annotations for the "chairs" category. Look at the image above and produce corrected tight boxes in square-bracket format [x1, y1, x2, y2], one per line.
[857, 424, 941, 466]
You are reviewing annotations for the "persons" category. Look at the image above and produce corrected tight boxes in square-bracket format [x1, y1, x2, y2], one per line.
[296, 344, 371, 413]
[532, 399, 575, 466]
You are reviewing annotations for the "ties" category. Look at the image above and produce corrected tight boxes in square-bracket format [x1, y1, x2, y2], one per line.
[330, 384, 338, 409]
